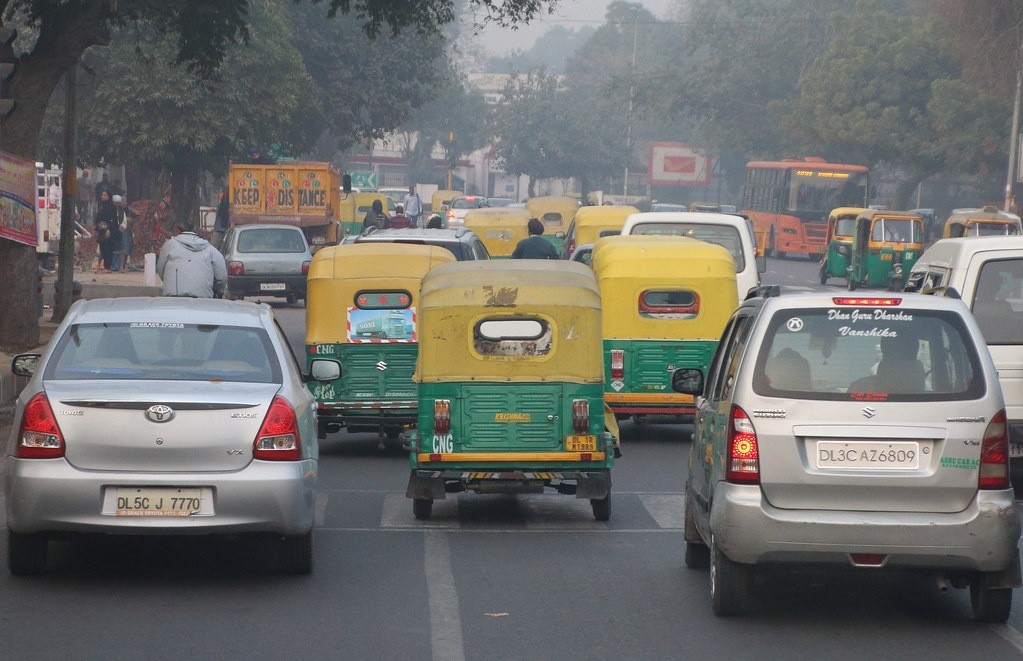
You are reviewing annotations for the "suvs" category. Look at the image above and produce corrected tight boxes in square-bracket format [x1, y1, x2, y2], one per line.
[355, 226, 492, 261]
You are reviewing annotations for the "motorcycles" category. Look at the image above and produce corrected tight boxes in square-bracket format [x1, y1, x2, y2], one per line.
[302, 241, 462, 446]
[527, 196, 582, 257]
[576, 206, 643, 255]
[463, 207, 532, 259]
[847, 208, 926, 292]
[943, 208, 1022, 238]
[818, 207, 863, 283]
[590, 238, 739, 424]
[432, 189, 465, 225]
[339, 191, 388, 242]
[409, 257, 616, 524]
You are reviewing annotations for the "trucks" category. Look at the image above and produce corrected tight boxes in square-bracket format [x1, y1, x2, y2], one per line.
[36, 163, 62, 269]
[226, 162, 352, 253]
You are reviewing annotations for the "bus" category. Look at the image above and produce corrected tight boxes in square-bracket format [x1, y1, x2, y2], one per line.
[742, 156, 870, 261]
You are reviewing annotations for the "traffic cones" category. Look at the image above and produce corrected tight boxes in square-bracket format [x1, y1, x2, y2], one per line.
[99, 258, 104, 271]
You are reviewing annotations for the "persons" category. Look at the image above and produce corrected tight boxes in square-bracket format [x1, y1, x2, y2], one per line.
[247, 148, 273, 164]
[94, 172, 138, 273]
[209, 188, 228, 249]
[511, 217, 560, 260]
[359, 199, 389, 235]
[403, 185, 423, 224]
[426, 213, 442, 228]
[479, 197, 488, 208]
[76, 171, 92, 224]
[387, 205, 418, 229]
[155, 221, 228, 299]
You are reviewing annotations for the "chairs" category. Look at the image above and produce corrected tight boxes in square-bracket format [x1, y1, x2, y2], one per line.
[93, 326, 143, 365]
[209, 329, 263, 366]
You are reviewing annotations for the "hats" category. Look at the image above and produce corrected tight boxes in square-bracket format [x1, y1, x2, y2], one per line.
[112, 195, 122, 203]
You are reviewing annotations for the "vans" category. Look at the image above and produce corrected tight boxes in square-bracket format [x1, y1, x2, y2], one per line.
[904, 235, 1023, 467]
[621, 213, 763, 304]
[379, 187, 419, 223]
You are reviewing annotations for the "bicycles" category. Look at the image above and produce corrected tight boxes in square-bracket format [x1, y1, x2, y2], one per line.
[128, 210, 210, 272]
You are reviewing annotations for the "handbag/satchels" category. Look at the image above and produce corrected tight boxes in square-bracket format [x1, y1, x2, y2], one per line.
[118, 211, 128, 232]
[97, 229, 110, 241]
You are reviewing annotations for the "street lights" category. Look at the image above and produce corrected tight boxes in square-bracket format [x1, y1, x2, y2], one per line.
[52, 59, 97, 323]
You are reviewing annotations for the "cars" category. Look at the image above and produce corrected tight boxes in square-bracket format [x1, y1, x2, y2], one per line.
[441, 195, 489, 229]
[487, 198, 527, 208]
[339, 235, 357, 245]
[690, 203, 721, 214]
[555, 217, 575, 259]
[387, 197, 398, 220]
[650, 204, 689, 215]
[221, 226, 312, 306]
[722, 206, 736, 215]
[571, 245, 593, 268]
[5, 294, 344, 581]
[671, 284, 1019, 624]
